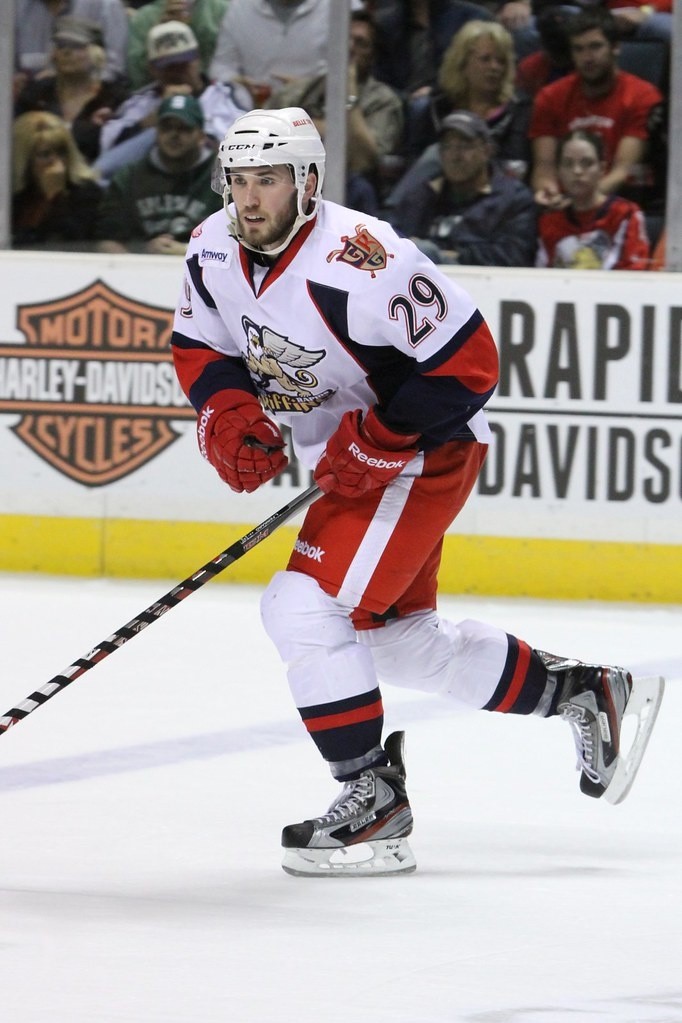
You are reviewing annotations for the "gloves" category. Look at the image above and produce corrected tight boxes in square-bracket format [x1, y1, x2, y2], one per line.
[316, 403, 422, 497]
[194, 389, 291, 494]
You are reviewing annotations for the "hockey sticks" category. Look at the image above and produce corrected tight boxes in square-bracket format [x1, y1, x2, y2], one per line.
[0, 474, 338, 735]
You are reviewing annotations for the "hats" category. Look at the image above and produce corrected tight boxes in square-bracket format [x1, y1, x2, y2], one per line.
[51, 16, 106, 45]
[157, 93, 206, 127]
[437, 110, 490, 148]
[146, 20, 199, 67]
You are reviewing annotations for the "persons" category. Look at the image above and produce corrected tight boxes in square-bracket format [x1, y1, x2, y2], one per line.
[346, 0, 673, 271]
[10, 0, 331, 254]
[171, 107, 664, 877]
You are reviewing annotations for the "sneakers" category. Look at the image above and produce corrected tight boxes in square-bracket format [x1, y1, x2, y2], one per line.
[281, 730, 418, 877]
[533, 649, 665, 805]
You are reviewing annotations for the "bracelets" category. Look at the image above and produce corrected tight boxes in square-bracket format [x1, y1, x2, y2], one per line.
[347, 96, 359, 110]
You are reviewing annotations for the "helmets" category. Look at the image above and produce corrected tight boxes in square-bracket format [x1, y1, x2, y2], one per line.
[210, 108, 327, 222]
